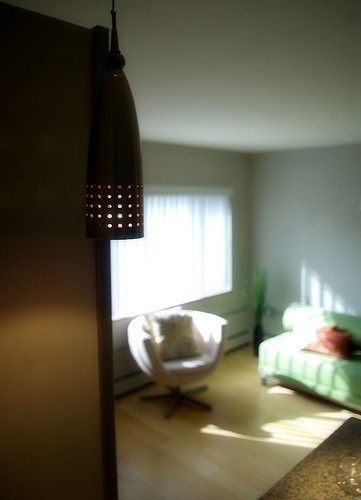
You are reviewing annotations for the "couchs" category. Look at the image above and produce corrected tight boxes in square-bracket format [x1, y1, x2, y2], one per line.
[258, 301, 361, 415]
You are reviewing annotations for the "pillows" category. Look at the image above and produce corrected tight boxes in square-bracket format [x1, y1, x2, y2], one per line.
[289, 309, 324, 354]
[146, 314, 202, 362]
[304, 323, 354, 360]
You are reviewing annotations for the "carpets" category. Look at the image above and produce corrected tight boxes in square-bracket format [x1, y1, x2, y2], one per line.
[257, 416, 361, 500]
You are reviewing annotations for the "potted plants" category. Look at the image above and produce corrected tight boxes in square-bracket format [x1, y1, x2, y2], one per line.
[249, 266, 268, 358]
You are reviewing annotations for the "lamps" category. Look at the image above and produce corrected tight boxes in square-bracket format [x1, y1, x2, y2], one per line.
[84, 0, 143, 240]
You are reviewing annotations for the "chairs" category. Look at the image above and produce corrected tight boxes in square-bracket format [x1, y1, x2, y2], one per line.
[126, 306, 229, 421]
